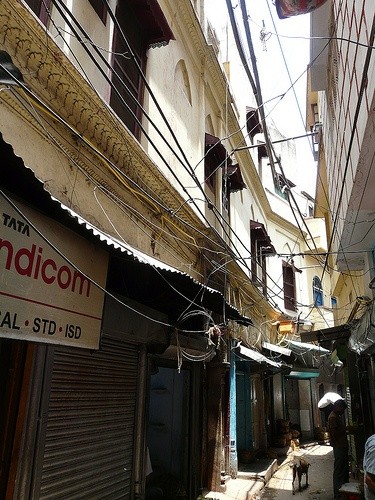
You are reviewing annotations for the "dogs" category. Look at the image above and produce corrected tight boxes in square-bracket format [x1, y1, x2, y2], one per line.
[290, 459, 311, 495]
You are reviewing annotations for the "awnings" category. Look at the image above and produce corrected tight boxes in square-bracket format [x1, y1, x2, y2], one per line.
[282, 260, 302, 273]
[277, 173, 296, 188]
[250, 220, 277, 257]
[205, 133, 232, 167]
[222, 163, 247, 193]
[301, 322, 353, 342]
[118, 0, 176, 50]
[246, 106, 263, 137]
[258, 139, 268, 157]
[236, 340, 330, 380]
[0, 102, 256, 328]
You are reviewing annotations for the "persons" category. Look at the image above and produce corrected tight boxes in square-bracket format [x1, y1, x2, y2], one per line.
[364, 434, 375, 500]
[327, 399, 356, 500]
[319, 402, 334, 446]
